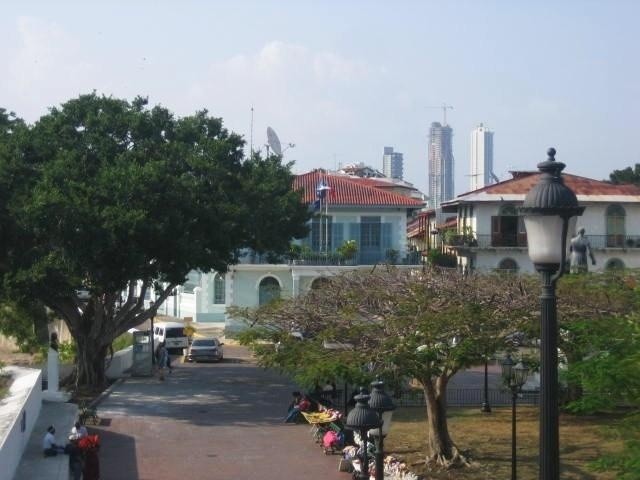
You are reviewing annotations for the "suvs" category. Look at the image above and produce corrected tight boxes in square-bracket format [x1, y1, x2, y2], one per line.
[120, 295, 146, 314]
[76, 287, 92, 301]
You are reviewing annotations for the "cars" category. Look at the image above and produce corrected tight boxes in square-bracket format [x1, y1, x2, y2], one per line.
[189, 335, 225, 363]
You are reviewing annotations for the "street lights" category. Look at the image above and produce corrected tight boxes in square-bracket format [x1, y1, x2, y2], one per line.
[480, 352, 493, 414]
[499, 350, 530, 480]
[342, 383, 384, 480]
[363, 377, 398, 480]
[430, 229, 439, 249]
[319, 186, 333, 263]
[516, 143, 586, 480]
[150, 298, 157, 367]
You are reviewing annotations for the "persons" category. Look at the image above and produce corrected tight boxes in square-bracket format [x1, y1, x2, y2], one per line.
[569, 225, 597, 273]
[158, 342, 168, 371]
[285, 392, 311, 425]
[84, 437, 100, 480]
[155, 343, 174, 369]
[70, 423, 88, 441]
[323, 380, 332, 392]
[41, 424, 66, 458]
[64, 435, 84, 480]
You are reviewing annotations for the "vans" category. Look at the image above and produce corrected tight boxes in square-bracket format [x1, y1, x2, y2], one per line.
[152, 321, 190, 353]
